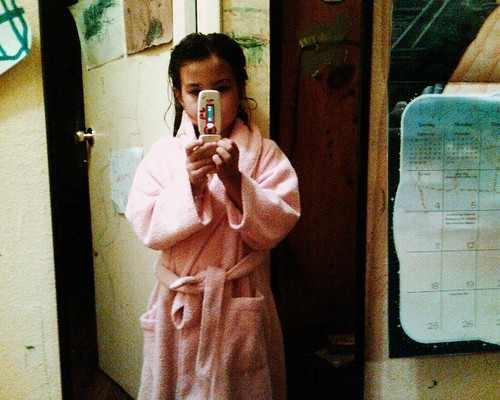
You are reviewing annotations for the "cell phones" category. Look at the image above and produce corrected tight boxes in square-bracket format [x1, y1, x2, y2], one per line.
[196, 90, 222, 144]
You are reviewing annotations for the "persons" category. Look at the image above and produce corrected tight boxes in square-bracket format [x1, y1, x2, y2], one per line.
[125, 30, 303, 400]
